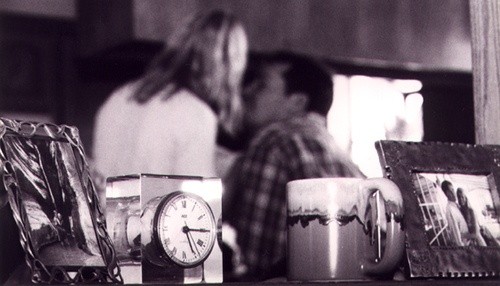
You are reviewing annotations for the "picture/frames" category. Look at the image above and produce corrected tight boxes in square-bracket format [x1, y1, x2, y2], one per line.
[375, 141, 499, 279]
[0, 117, 125, 286]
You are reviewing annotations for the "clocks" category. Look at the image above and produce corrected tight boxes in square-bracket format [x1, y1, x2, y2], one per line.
[142, 191, 217, 282]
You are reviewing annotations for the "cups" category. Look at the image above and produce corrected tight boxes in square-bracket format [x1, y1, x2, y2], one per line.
[286, 177, 407, 284]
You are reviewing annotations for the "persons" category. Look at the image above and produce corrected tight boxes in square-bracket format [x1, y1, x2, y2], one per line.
[218, 50, 374, 286]
[91, 5, 252, 186]
[456, 187, 487, 248]
[442, 180, 472, 249]
